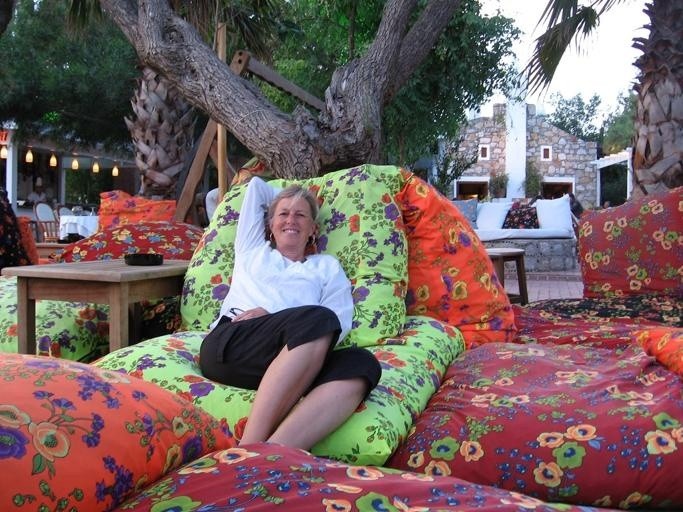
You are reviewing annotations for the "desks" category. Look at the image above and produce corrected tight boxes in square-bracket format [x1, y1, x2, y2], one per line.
[0, 257, 188, 360]
[59, 216, 99, 242]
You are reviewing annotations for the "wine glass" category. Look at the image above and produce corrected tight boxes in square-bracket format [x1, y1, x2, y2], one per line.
[89, 206, 96, 216]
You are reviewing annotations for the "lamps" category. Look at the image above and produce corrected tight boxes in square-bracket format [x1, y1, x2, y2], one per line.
[0, 145, 120, 177]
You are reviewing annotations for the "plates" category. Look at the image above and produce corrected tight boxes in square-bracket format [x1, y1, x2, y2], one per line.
[125, 252, 164, 266]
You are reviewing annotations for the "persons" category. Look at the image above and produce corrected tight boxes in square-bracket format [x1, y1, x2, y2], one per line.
[202, 139, 237, 226]
[196, 173, 384, 455]
[602, 200, 612, 209]
[25, 176, 47, 206]
[568, 193, 583, 219]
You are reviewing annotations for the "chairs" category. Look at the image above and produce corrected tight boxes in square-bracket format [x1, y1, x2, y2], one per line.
[33, 200, 75, 242]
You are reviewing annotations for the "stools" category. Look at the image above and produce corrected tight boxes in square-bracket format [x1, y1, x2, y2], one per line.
[484, 249, 530, 305]
